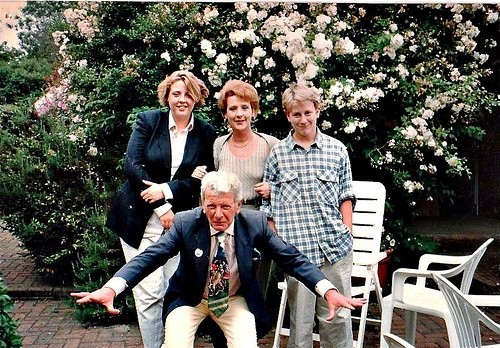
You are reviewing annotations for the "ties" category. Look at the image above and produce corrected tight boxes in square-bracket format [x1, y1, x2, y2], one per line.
[208, 232, 231, 318]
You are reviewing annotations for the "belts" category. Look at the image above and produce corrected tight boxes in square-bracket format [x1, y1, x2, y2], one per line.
[240, 199, 262, 209]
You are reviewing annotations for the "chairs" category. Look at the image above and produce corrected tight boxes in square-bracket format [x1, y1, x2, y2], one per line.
[431, 271, 500, 348]
[382, 333, 417, 348]
[272, 181, 387, 348]
[380, 238, 494, 348]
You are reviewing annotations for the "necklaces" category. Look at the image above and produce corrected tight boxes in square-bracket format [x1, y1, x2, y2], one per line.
[231, 134, 254, 148]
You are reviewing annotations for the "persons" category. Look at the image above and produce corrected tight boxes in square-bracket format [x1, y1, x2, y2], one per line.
[105, 70, 218, 348]
[191, 79, 280, 348]
[70, 171, 368, 348]
[260, 84, 358, 348]
